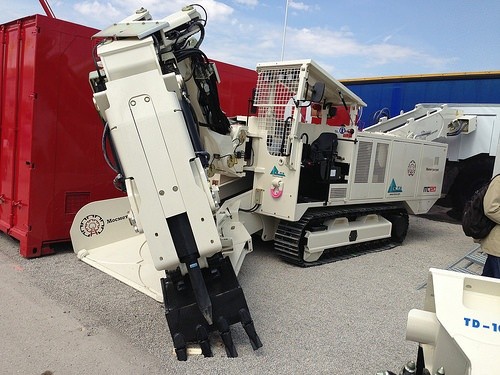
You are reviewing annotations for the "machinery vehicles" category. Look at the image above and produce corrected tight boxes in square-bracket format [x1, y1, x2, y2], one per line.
[67, 8, 453, 360]
